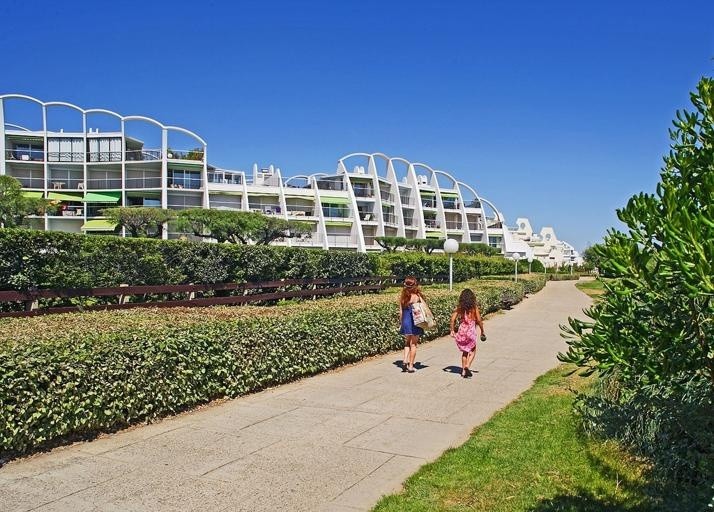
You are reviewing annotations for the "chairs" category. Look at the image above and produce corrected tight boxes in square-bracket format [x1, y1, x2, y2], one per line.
[364, 215, 370, 221]
[7, 151, 16, 160]
[22, 155, 30, 160]
[78, 182, 84, 189]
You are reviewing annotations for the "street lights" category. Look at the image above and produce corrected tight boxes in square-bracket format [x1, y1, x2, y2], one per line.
[528, 257, 533, 273]
[544, 260, 547, 273]
[512, 252, 520, 283]
[444, 238, 460, 292]
[569, 262, 573, 275]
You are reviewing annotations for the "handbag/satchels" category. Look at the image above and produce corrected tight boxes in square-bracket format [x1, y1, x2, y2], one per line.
[411, 299, 437, 331]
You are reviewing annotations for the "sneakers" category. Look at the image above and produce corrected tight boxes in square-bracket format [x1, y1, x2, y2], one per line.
[462, 366, 472, 378]
[403, 362, 417, 373]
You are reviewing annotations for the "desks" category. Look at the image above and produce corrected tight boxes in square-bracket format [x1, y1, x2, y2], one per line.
[52, 182, 65, 189]
[287, 211, 305, 216]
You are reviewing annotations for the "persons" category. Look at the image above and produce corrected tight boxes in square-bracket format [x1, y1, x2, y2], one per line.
[399, 275, 436, 372]
[450, 289, 485, 377]
